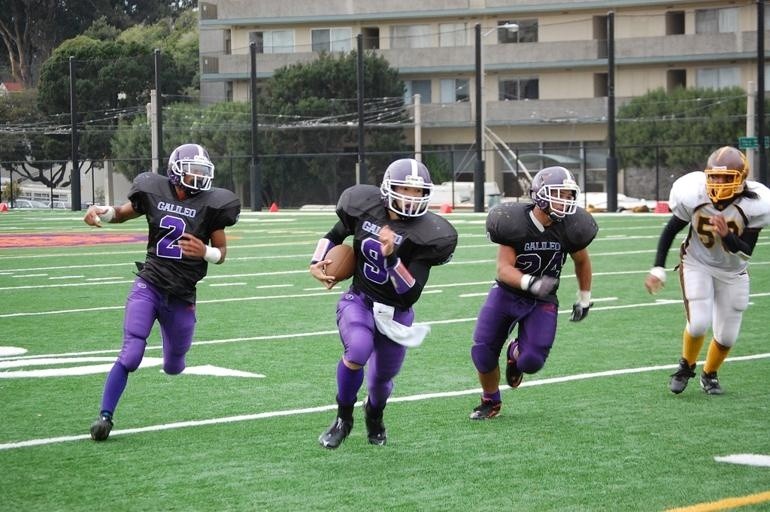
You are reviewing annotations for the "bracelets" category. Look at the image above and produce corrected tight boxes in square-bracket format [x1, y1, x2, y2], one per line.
[204, 245, 222, 265]
[93, 204, 114, 224]
[576, 290, 591, 310]
[520, 273, 533, 292]
[649, 266, 666, 282]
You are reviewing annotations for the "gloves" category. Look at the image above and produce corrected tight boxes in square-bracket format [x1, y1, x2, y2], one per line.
[519, 272, 560, 301]
[567, 297, 593, 322]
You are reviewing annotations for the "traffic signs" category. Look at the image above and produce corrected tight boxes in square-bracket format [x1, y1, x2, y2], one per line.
[738, 136, 770, 149]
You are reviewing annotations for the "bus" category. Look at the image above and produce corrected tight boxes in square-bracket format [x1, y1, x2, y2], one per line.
[0, 177, 72, 203]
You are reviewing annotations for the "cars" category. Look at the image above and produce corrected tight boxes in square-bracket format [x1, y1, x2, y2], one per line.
[1, 199, 122, 209]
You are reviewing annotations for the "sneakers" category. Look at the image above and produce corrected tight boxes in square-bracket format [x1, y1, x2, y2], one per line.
[317, 409, 355, 450]
[670, 356, 696, 395]
[364, 408, 388, 447]
[506, 337, 524, 388]
[700, 368, 722, 395]
[90, 413, 114, 442]
[468, 397, 503, 420]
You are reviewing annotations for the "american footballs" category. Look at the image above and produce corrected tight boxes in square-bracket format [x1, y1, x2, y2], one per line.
[323, 244, 356, 282]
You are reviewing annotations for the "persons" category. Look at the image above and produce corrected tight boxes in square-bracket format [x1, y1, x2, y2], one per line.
[469, 165, 599, 419]
[81, 144, 241, 440]
[310, 159, 458, 448]
[644, 145, 769, 396]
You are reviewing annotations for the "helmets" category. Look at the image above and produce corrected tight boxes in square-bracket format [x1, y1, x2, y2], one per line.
[529, 165, 580, 224]
[702, 144, 751, 205]
[164, 142, 215, 198]
[376, 158, 438, 223]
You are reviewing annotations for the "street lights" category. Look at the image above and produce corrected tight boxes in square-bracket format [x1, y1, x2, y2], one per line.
[474, 23, 520, 161]
